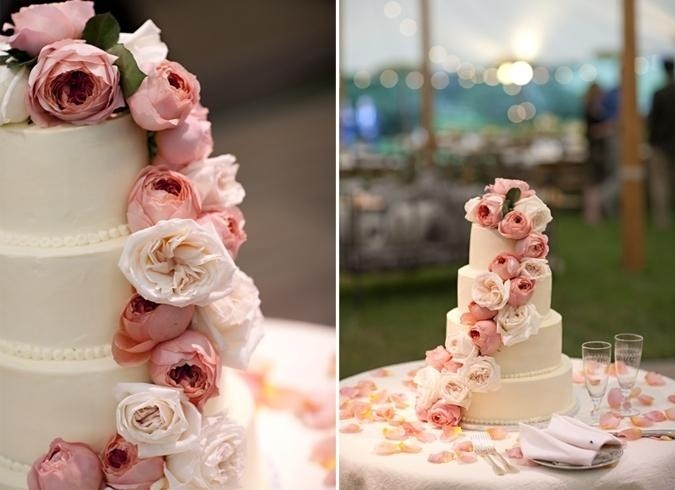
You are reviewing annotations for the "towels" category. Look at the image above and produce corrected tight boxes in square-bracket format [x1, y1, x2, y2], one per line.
[516, 414, 624, 467]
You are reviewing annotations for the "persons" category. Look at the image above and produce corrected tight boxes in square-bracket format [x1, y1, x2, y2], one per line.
[578, 81, 611, 228]
[643, 56, 675, 230]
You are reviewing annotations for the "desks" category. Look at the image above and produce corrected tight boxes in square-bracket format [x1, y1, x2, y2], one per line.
[340, 359, 674, 490]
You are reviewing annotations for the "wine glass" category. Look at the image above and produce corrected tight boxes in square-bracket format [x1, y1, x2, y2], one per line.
[581, 334, 644, 426]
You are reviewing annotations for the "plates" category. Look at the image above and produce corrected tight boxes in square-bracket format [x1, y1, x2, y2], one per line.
[528, 448, 623, 472]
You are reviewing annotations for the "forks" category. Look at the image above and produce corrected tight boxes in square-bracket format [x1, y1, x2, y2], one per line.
[470, 433, 518, 477]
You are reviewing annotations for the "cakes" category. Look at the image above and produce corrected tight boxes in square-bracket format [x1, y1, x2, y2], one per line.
[444, 224, 574, 425]
[0, 111, 150, 490]
